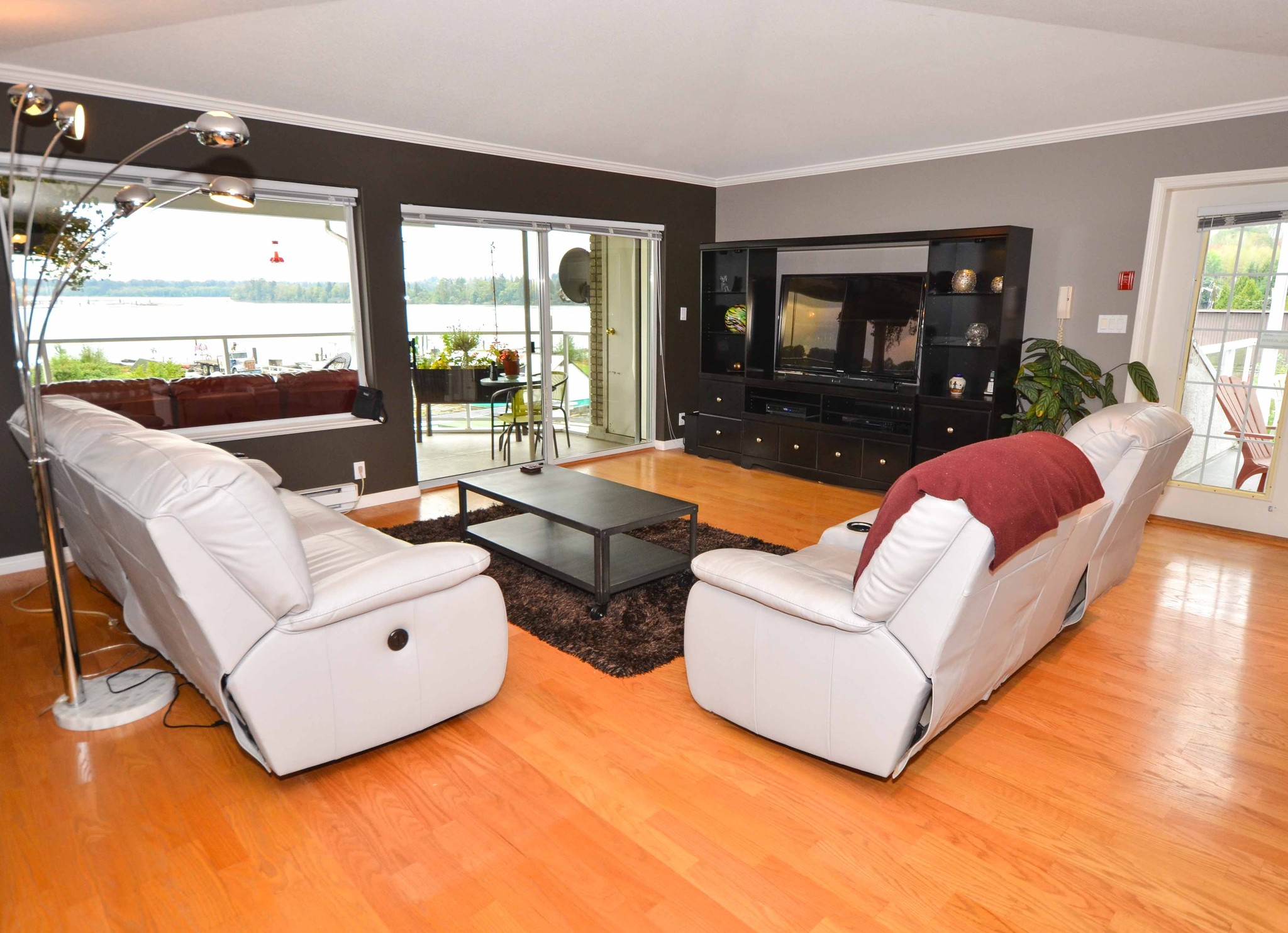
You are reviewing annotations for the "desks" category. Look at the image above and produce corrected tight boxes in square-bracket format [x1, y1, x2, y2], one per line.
[479, 375, 542, 442]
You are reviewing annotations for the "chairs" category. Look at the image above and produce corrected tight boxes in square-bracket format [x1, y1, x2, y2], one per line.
[322, 352, 352, 369]
[1213, 375, 1273, 493]
[491, 371, 570, 466]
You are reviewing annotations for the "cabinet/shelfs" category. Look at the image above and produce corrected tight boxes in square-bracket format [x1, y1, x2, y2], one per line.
[682, 224, 1035, 492]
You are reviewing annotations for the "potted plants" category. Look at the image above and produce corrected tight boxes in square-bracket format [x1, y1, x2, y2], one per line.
[411, 321, 528, 443]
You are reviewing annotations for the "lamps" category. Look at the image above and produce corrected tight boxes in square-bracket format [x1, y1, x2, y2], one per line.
[0, 79, 256, 730]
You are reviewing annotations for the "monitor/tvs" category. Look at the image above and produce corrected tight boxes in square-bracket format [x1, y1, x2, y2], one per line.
[773, 271, 927, 391]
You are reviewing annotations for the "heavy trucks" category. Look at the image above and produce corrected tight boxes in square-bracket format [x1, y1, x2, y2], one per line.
[193, 351, 262, 376]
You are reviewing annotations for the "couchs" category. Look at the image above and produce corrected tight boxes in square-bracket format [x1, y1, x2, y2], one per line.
[682, 403, 1196, 781]
[6, 391, 509, 779]
[30, 367, 360, 431]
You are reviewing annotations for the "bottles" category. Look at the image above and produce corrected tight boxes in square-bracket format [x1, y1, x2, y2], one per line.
[948, 374, 967, 395]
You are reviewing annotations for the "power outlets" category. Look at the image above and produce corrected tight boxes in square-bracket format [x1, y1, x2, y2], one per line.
[679, 412, 685, 426]
[353, 461, 366, 480]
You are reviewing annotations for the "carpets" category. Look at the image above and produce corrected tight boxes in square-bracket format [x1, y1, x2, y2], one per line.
[375, 505, 798, 679]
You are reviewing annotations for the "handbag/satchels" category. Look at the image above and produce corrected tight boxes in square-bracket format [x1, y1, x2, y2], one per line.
[350, 385, 388, 424]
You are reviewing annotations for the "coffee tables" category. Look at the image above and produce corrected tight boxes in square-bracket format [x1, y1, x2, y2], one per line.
[458, 464, 698, 621]
